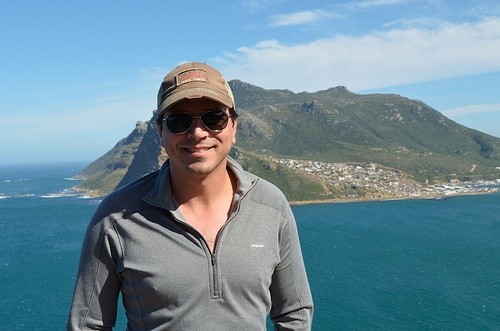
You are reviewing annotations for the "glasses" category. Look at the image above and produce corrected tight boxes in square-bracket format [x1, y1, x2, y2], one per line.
[163, 111, 236, 135]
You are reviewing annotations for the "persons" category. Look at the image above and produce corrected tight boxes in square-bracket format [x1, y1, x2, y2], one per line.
[66, 62, 314, 331]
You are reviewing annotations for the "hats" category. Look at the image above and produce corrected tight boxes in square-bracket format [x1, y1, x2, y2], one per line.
[156, 61, 235, 119]
